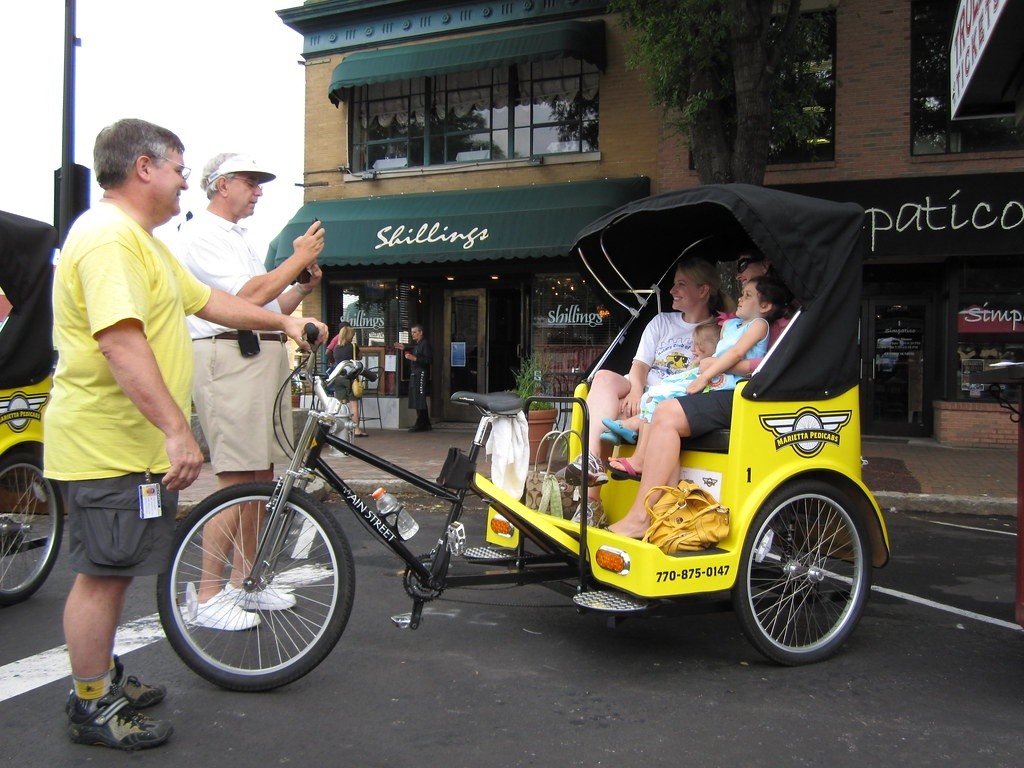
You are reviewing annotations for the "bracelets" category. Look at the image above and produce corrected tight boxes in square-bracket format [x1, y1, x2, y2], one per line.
[293, 283, 313, 296]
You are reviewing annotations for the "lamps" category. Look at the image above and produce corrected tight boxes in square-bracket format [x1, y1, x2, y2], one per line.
[805, 133, 831, 146]
[804, 99, 826, 113]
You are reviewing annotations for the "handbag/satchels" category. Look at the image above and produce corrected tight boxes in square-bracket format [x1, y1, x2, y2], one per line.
[526, 429, 582, 521]
[352, 380, 363, 397]
[643, 480, 729, 554]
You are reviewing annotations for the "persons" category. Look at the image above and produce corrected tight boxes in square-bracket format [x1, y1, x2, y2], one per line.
[141, 487, 155, 497]
[393, 324, 433, 433]
[40, 119, 325, 751]
[561, 241, 793, 542]
[325, 321, 369, 437]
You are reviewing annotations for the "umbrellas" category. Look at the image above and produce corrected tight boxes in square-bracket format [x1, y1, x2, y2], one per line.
[956, 305, 1024, 341]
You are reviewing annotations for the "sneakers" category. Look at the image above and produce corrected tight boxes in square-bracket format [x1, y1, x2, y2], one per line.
[571, 499, 608, 530]
[64, 682, 174, 751]
[110, 654, 164, 709]
[565, 449, 608, 487]
[225, 582, 296, 610]
[186, 589, 262, 632]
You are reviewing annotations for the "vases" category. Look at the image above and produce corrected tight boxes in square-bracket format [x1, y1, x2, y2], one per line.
[291, 393, 300, 407]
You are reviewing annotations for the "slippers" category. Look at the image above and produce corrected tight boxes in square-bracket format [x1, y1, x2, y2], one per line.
[353, 430, 370, 437]
[602, 527, 643, 541]
[606, 457, 642, 482]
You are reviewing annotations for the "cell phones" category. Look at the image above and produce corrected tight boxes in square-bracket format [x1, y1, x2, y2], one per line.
[312, 218, 319, 235]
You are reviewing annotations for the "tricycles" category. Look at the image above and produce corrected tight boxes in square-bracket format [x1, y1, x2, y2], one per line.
[0, 209, 60, 611]
[156, 179, 894, 692]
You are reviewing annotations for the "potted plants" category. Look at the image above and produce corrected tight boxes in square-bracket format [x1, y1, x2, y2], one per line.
[511, 355, 558, 465]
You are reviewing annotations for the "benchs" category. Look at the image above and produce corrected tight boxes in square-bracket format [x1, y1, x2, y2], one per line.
[603, 310, 794, 452]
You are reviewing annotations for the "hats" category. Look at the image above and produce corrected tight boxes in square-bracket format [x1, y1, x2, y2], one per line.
[205, 153, 277, 184]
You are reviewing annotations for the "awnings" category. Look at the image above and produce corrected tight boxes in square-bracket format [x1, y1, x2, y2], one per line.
[328, 18, 606, 110]
[263, 176, 654, 286]
[761, 172, 1023, 304]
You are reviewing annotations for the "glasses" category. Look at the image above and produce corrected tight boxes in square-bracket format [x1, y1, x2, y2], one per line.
[735, 258, 762, 273]
[160, 154, 192, 181]
[225, 176, 264, 191]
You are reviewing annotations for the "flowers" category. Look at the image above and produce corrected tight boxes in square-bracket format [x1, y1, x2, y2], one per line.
[290, 369, 303, 395]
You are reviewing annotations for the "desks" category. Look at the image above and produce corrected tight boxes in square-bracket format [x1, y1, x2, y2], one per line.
[371, 157, 417, 169]
[457, 149, 490, 162]
[546, 141, 589, 155]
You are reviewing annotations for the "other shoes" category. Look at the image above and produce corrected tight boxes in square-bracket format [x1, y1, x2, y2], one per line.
[408, 418, 432, 433]
[603, 418, 639, 446]
[601, 431, 629, 447]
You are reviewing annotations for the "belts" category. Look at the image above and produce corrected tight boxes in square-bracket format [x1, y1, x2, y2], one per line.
[206, 333, 288, 343]
